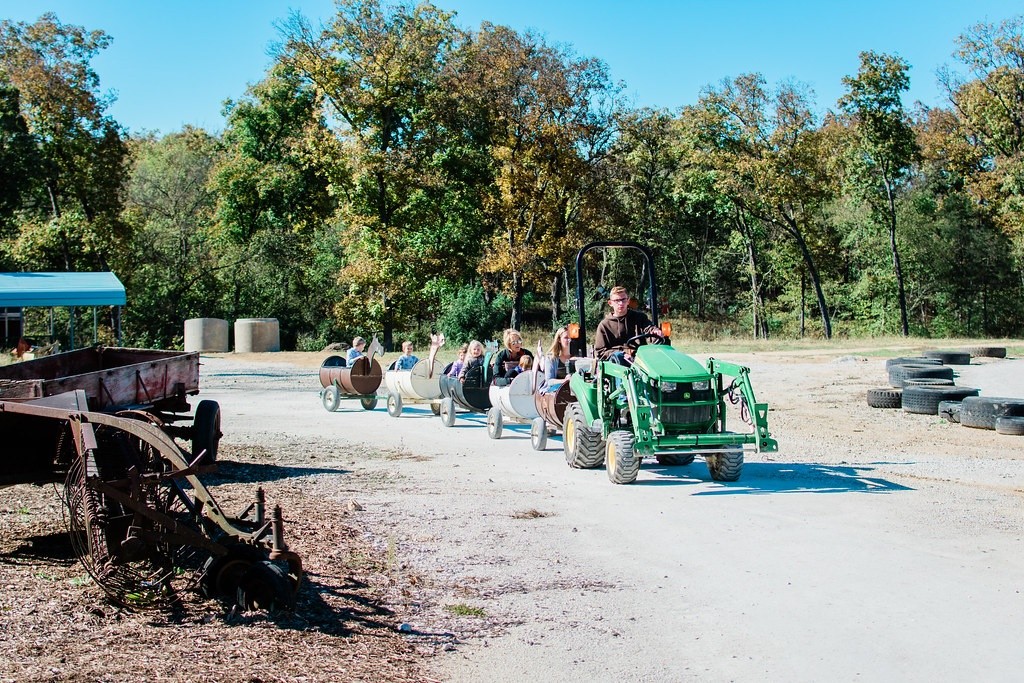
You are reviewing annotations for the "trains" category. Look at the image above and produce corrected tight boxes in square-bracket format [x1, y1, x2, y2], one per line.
[318, 240, 779, 485]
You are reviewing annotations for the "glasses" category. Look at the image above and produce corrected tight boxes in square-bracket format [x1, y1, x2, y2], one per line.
[613, 297, 628, 304]
[510, 338, 522, 345]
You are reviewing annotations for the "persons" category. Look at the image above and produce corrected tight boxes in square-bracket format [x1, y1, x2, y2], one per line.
[458, 340, 494, 383]
[394, 341, 419, 372]
[504, 355, 533, 383]
[492, 329, 534, 386]
[537, 326, 572, 396]
[594, 286, 664, 402]
[346, 336, 366, 368]
[447, 346, 467, 378]
